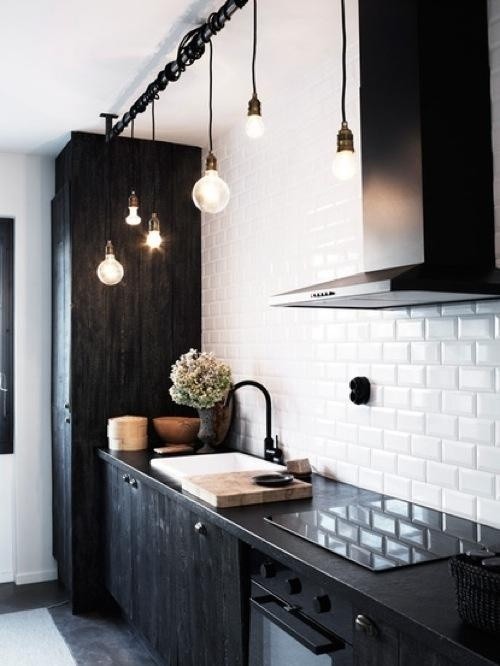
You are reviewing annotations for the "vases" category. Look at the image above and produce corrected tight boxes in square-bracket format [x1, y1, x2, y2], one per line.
[196, 389, 234, 452]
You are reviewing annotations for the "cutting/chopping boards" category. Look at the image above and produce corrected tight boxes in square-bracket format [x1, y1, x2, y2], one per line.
[178, 469, 311, 509]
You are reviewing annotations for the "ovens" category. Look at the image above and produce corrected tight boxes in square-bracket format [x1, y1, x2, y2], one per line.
[240, 580, 358, 666]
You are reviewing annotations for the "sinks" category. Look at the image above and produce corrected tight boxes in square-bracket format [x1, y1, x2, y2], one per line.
[150, 451, 285, 476]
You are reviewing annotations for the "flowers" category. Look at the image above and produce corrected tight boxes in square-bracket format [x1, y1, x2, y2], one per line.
[169, 348, 232, 411]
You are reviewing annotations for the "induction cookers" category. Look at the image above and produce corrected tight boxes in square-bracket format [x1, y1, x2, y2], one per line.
[251, 498, 490, 645]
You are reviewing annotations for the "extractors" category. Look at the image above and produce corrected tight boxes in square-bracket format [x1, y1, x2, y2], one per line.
[268, 0, 500, 311]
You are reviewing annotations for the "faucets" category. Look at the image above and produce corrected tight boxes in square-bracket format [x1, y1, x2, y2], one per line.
[224, 379, 272, 436]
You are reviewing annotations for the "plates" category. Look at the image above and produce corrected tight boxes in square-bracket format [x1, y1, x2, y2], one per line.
[253, 473, 294, 485]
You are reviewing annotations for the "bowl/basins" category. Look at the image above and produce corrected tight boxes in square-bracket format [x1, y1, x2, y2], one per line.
[152, 415, 200, 445]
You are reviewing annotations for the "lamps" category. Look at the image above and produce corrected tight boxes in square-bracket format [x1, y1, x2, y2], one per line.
[145, 98, 163, 249]
[123, 114, 144, 227]
[192, 41, 229, 214]
[243, 1, 267, 139]
[96, 131, 125, 287]
[331, 1, 356, 179]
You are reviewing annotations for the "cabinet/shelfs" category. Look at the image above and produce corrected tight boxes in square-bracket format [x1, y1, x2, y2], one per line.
[352, 604, 461, 666]
[93, 453, 243, 666]
[50, 182, 73, 612]
